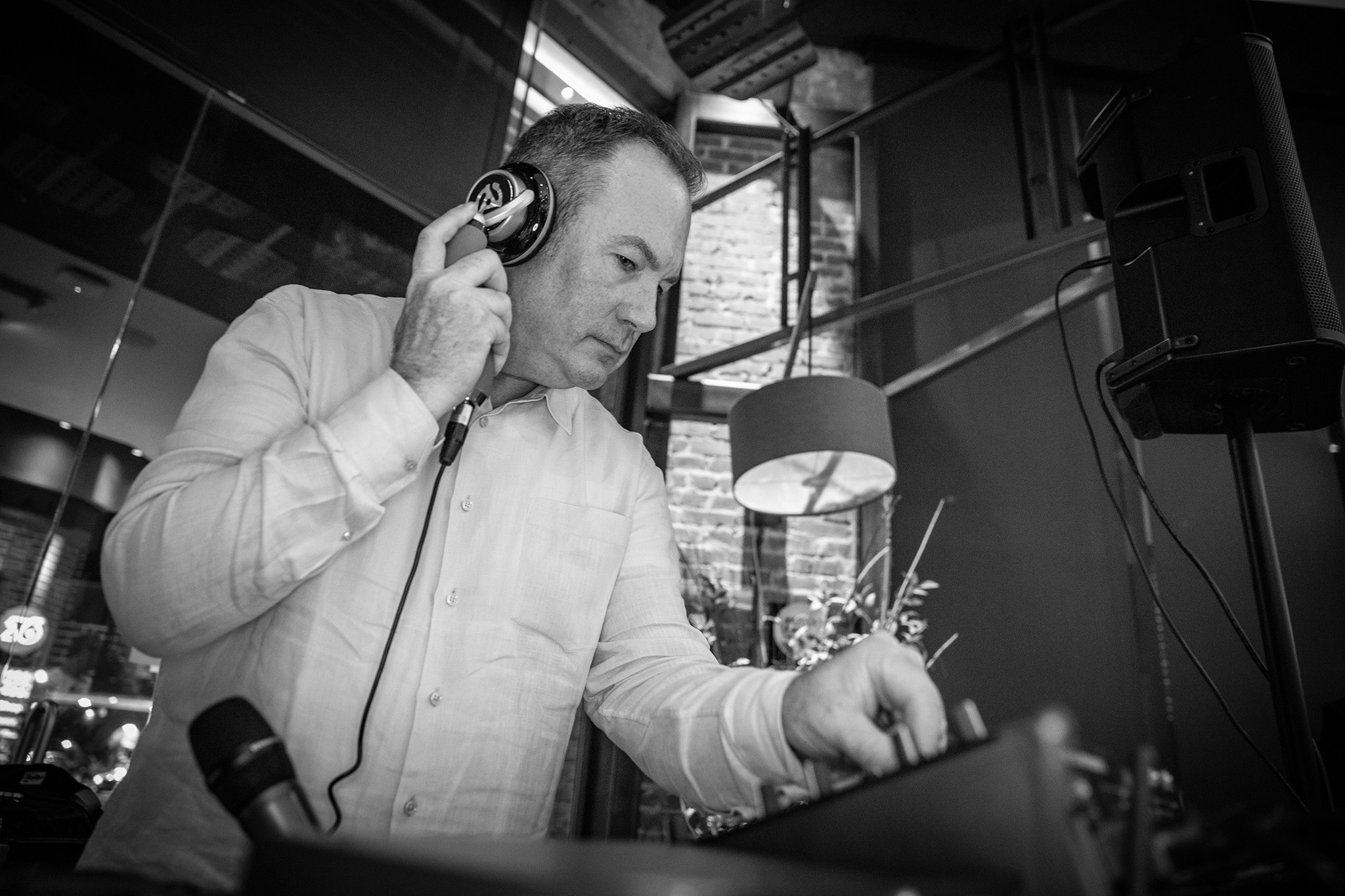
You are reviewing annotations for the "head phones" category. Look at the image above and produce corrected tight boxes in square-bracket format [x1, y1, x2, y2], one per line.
[444, 156, 561, 407]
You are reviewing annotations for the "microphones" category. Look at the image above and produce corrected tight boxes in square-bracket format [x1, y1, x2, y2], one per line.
[181, 691, 316, 851]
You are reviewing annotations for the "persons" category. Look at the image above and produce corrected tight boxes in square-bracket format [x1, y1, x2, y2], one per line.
[73, 105, 949, 896]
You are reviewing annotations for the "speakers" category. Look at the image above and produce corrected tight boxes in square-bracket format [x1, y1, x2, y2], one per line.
[1072, 31, 1344, 441]
